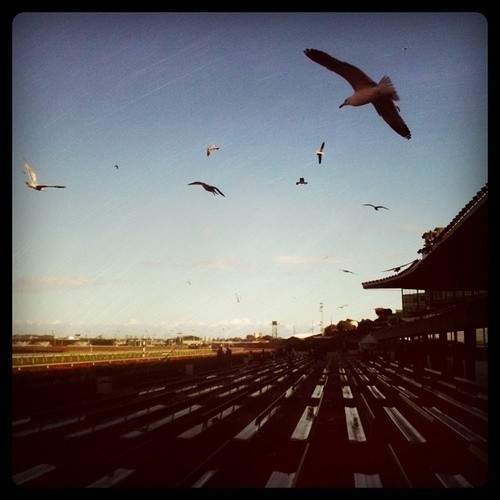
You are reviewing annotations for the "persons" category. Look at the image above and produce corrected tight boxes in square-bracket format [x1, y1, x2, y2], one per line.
[217, 347, 224, 361]
[248, 348, 275, 362]
[226, 348, 232, 364]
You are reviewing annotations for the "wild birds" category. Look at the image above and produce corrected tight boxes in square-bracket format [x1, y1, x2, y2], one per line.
[302, 45, 413, 141]
[235, 292, 242, 303]
[361, 202, 389, 212]
[113, 164, 119, 170]
[316, 141, 326, 165]
[206, 144, 220, 157]
[186, 279, 193, 286]
[21, 155, 67, 192]
[295, 177, 308, 186]
[187, 180, 225, 197]
[380, 261, 413, 274]
[337, 268, 356, 275]
[335, 304, 348, 310]
